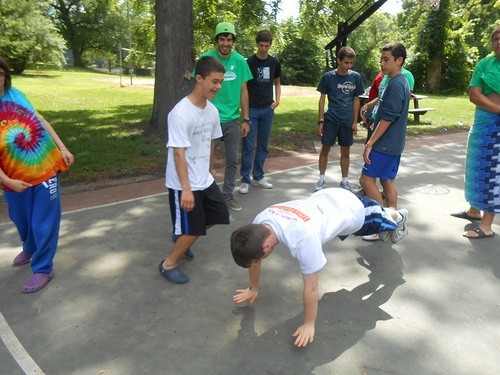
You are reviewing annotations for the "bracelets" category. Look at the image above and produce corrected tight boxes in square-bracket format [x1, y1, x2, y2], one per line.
[317, 120, 324, 125]
[243, 117, 250, 123]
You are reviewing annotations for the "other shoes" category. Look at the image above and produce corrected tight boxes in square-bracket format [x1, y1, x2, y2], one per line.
[339, 180, 352, 190]
[362, 232, 381, 240]
[314, 178, 327, 192]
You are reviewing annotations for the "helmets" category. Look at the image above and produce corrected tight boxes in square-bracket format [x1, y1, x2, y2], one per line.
[214, 22, 237, 38]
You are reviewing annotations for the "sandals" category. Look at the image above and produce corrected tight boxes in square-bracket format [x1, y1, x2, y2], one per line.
[159, 260, 189, 284]
[22, 270, 55, 293]
[13, 250, 32, 265]
[183, 248, 194, 260]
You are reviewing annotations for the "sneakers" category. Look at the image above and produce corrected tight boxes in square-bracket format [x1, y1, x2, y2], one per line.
[392, 208, 409, 243]
[224, 195, 242, 212]
[380, 231, 390, 242]
[251, 178, 274, 188]
[238, 183, 250, 194]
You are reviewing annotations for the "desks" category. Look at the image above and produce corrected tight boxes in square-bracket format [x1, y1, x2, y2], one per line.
[409, 94, 428, 122]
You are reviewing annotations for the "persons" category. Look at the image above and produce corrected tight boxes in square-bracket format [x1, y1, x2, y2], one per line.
[451, 26, 499, 238]
[369, 70, 385, 101]
[238, 29, 282, 194]
[158, 56, 232, 285]
[0, 56, 75, 294]
[316, 45, 366, 192]
[189, 22, 250, 211]
[359, 42, 411, 240]
[230, 187, 408, 348]
[357, 58, 416, 197]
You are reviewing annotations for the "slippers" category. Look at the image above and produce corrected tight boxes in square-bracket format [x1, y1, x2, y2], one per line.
[463, 228, 495, 239]
[450, 211, 482, 221]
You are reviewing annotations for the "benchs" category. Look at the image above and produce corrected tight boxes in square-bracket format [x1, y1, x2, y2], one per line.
[407, 108, 436, 123]
